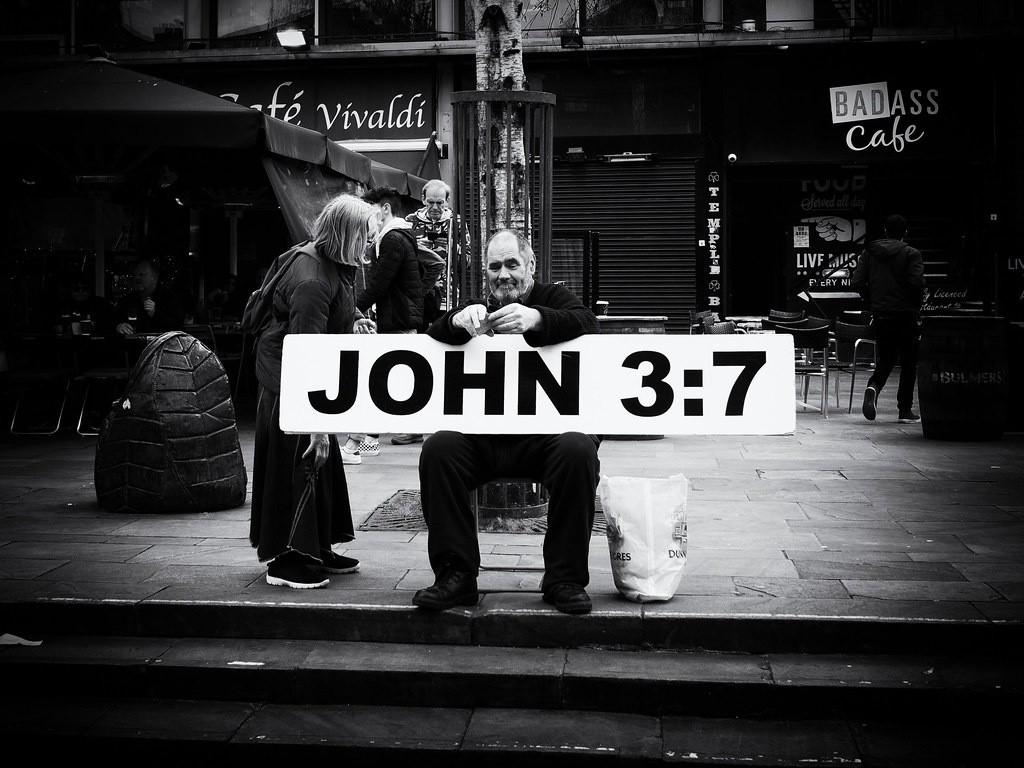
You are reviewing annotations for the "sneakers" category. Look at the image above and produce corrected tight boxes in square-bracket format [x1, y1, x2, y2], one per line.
[266, 541, 360, 589]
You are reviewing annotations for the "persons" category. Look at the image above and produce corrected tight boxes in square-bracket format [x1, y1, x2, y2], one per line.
[139, 165, 189, 259]
[16, 260, 182, 433]
[412, 228, 600, 615]
[338, 179, 472, 465]
[850, 215, 926, 420]
[249, 195, 381, 589]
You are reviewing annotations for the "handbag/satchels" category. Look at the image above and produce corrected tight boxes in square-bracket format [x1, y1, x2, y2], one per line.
[600, 472, 689, 601]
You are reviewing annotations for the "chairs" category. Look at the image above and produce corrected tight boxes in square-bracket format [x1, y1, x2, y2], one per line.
[9, 325, 249, 438]
[687, 307, 879, 413]
[474, 478, 546, 594]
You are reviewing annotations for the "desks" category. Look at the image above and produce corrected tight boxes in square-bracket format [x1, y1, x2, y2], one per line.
[724, 315, 769, 332]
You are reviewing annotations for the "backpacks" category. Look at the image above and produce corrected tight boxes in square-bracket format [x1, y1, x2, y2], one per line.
[239, 240, 339, 338]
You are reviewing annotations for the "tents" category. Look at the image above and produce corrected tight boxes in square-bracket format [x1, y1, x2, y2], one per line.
[0, 62, 425, 404]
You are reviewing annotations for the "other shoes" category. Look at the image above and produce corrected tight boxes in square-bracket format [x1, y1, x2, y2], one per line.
[861, 385, 877, 420]
[544, 583, 591, 614]
[411, 567, 478, 610]
[898, 408, 921, 424]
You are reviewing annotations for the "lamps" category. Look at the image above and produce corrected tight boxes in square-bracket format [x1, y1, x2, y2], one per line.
[275, 26, 310, 52]
[567, 146, 587, 162]
[560, 31, 582, 48]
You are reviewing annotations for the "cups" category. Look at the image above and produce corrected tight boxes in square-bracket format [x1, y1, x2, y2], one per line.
[71, 323, 81, 335]
[128, 317, 137, 334]
[80, 320, 90, 336]
[143, 296, 150, 308]
[596, 301, 609, 316]
